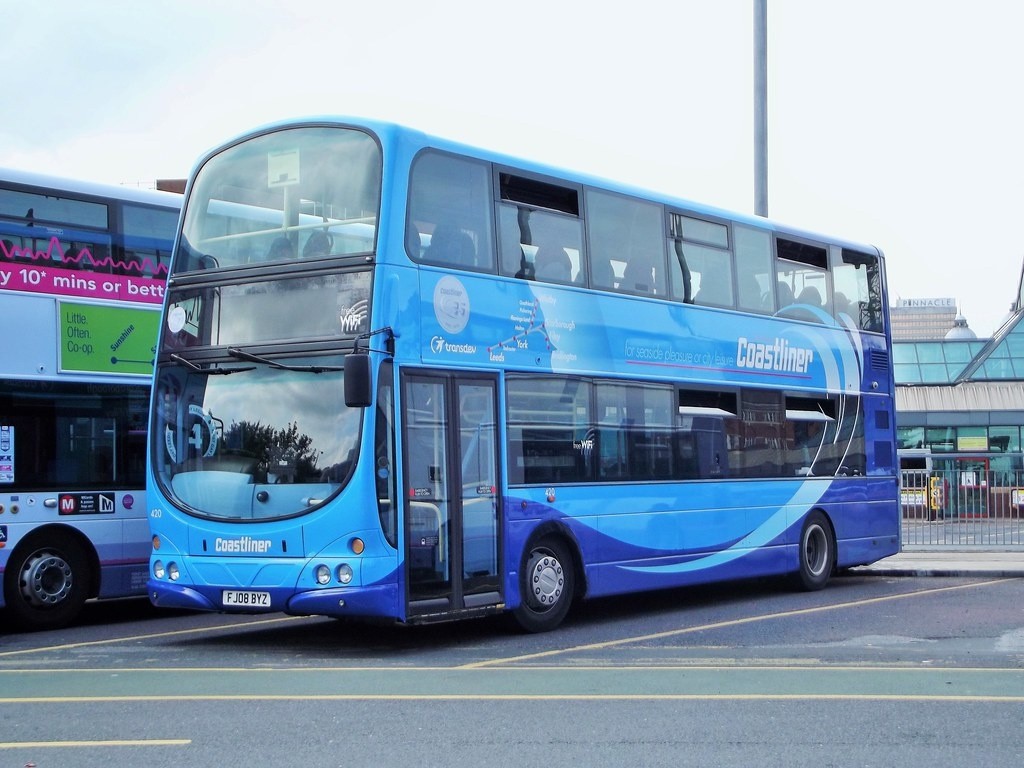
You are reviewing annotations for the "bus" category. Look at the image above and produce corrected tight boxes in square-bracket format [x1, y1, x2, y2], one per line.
[138, 114, 902, 636]
[0, 162, 538, 628]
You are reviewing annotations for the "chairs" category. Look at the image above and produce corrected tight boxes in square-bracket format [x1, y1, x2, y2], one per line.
[422, 216, 462, 267]
[795, 286, 822, 321]
[478, 242, 526, 276]
[533, 240, 573, 282]
[60, 248, 86, 270]
[265, 237, 294, 262]
[574, 250, 615, 289]
[406, 221, 422, 258]
[0, 239, 16, 264]
[153, 266, 168, 281]
[126, 256, 144, 277]
[303, 231, 334, 258]
[824, 292, 849, 326]
[850, 300, 868, 331]
[458, 233, 476, 267]
[762, 281, 798, 316]
[618, 254, 655, 294]
[34, 254, 54, 267]
